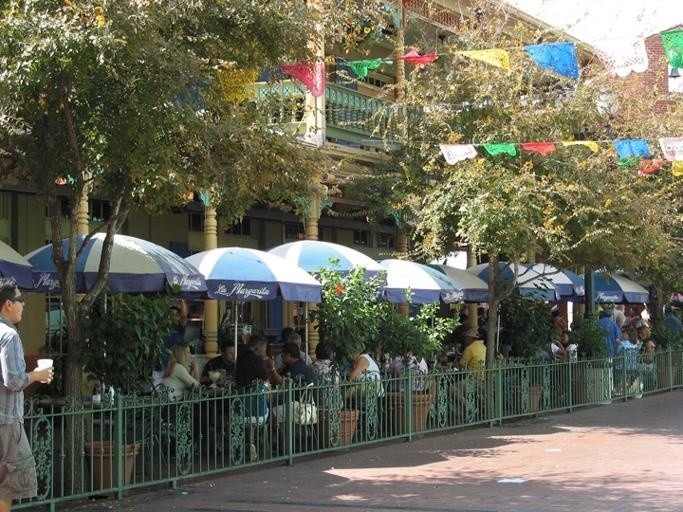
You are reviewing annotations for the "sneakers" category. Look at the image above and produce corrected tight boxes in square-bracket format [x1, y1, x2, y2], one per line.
[250, 442, 258, 461]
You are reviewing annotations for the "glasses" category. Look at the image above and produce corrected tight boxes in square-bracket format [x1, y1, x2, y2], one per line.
[9, 294, 24, 303]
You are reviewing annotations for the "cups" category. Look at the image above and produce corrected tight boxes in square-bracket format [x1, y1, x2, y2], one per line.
[37, 359, 53, 384]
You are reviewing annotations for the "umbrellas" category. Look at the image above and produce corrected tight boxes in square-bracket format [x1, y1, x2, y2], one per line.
[521, 261, 584, 304]
[0, 238, 35, 289]
[593, 269, 652, 306]
[23, 230, 208, 367]
[466, 259, 556, 351]
[264, 238, 389, 353]
[426, 262, 489, 307]
[376, 257, 464, 310]
[182, 245, 321, 360]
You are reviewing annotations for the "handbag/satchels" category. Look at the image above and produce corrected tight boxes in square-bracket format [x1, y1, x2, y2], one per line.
[292, 382, 318, 425]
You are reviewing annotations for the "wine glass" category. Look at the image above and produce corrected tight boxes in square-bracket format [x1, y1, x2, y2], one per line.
[208, 371, 221, 390]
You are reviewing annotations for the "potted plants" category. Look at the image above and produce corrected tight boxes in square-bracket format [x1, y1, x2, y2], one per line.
[496, 294, 680, 416]
[79, 281, 186, 496]
[303, 255, 465, 447]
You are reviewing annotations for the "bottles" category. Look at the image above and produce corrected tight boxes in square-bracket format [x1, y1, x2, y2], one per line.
[109, 387, 115, 401]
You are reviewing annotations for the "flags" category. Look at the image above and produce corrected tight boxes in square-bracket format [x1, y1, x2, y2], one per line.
[593, 34, 647, 78]
[437, 136, 683, 167]
[393, 50, 439, 69]
[456, 48, 511, 71]
[216, 66, 257, 105]
[520, 43, 581, 82]
[279, 62, 328, 100]
[660, 29, 683, 68]
[336, 57, 387, 81]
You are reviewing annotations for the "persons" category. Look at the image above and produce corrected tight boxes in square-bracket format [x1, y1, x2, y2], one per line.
[388, 319, 486, 420]
[149, 309, 386, 463]
[498, 307, 621, 407]
[0, 275, 55, 512]
[614, 300, 682, 389]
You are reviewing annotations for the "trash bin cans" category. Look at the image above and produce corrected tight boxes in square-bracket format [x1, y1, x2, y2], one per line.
[578, 361, 613, 407]
